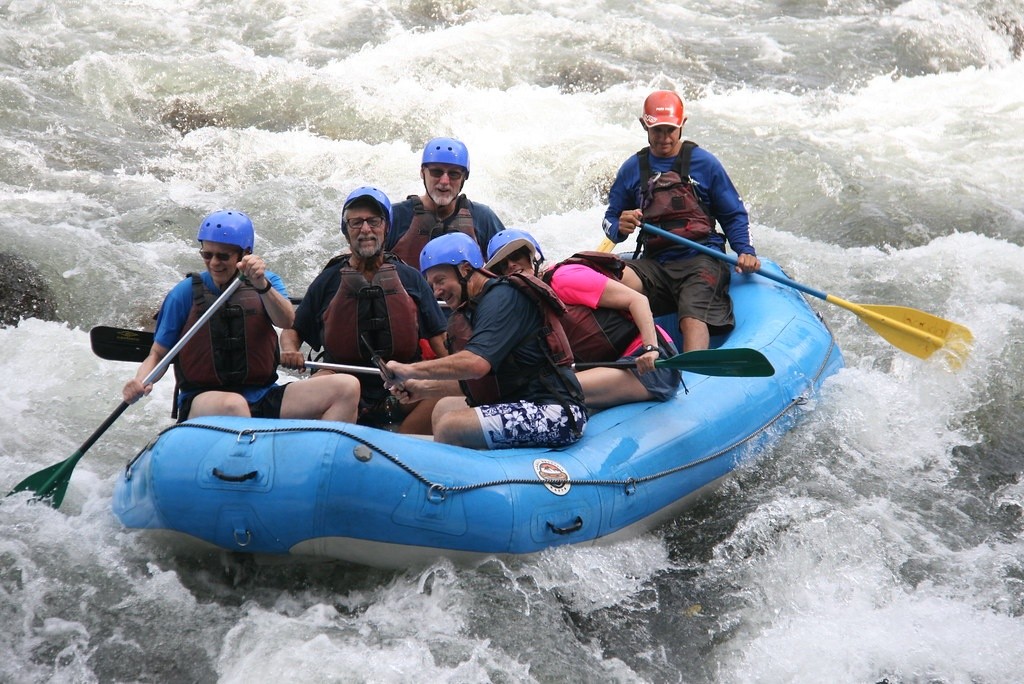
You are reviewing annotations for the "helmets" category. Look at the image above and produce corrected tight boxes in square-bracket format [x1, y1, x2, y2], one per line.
[419, 232, 484, 277]
[487, 229, 544, 261]
[197, 210, 254, 255]
[341, 187, 393, 239]
[421, 137, 470, 180]
[642, 91, 684, 128]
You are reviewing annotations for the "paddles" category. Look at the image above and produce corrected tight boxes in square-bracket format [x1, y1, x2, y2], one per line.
[596, 235, 620, 254]
[639, 221, 975, 372]
[1, 273, 249, 511]
[570, 348, 776, 378]
[358, 331, 415, 401]
[289, 294, 449, 307]
[88, 324, 385, 378]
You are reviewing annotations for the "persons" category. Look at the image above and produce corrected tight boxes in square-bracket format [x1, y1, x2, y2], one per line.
[483, 228, 681, 408]
[123, 210, 360, 425]
[384, 137, 505, 272]
[279, 187, 462, 433]
[380, 233, 587, 450]
[603, 90, 760, 354]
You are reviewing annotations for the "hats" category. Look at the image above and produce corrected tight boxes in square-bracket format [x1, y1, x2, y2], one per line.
[345, 194, 387, 218]
[483, 238, 536, 271]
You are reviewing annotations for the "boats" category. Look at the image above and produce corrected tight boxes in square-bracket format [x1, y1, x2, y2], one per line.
[113, 252, 852, 575]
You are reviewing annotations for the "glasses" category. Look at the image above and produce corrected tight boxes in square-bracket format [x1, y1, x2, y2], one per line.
[346, 217, 384, 229]
[425, 164, 465, 180]
[494, 248, 522, 273]
[199, 248, 240, 261]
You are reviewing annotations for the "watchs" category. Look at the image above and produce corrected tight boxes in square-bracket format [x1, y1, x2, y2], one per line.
[255, 277, 273, 294]
[644, 344, 661, 353]
